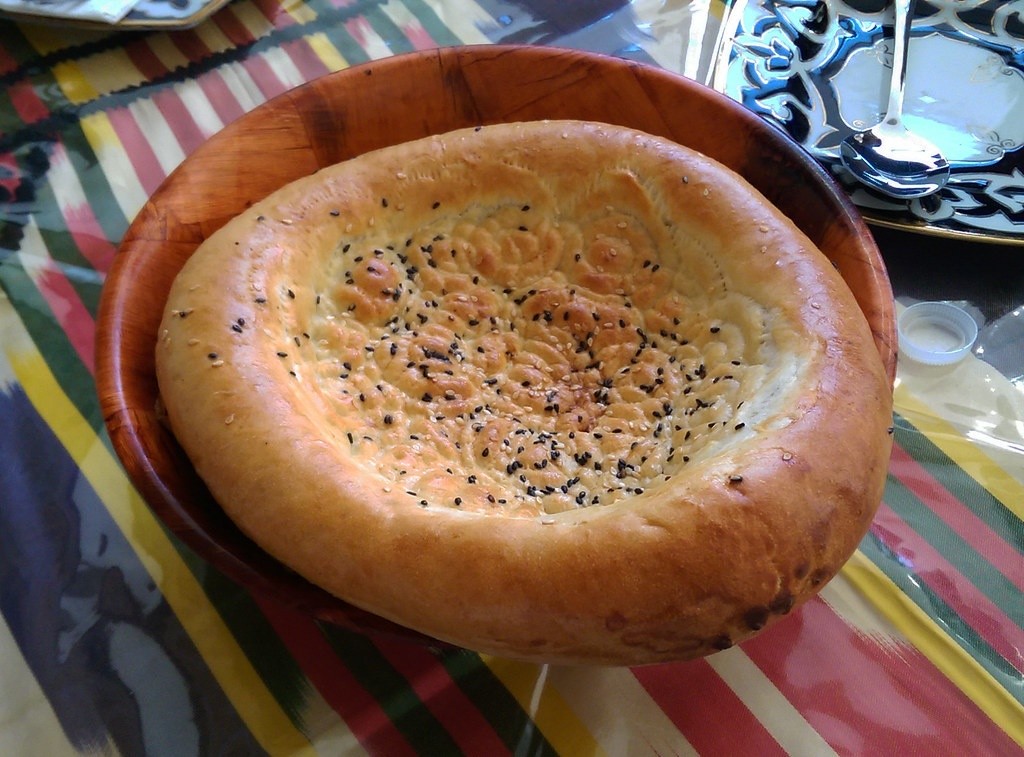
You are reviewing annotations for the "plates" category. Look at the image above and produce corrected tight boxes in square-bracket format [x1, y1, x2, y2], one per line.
[95, 44, 900, 648]
[685, 0, 1024, 244]
[0, 0, 230, 30]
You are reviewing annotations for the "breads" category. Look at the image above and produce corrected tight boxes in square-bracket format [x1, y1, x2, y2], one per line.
[152, 121, 891, 663]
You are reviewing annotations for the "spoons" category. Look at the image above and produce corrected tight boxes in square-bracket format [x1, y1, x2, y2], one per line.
[839, 0, 951, 196]
